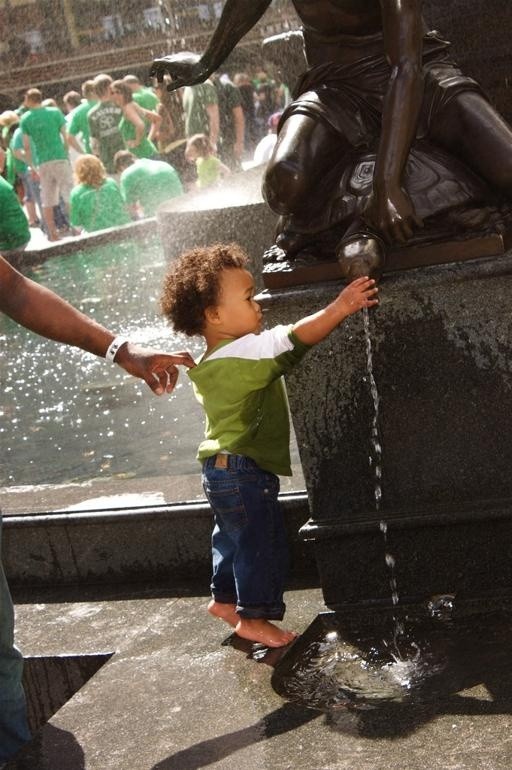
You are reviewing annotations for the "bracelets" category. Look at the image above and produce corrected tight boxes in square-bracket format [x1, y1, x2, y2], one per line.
[104, 336, 129, 365]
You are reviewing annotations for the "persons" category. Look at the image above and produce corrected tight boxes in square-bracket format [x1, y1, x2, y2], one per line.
[0, 64, 291, 248]
[114, 148, 183, 221]
[0, 175, 30, 249]
[0, 254, 199, 770]
[20, 88, 81, 241]
[185, 134, 231, 189]
[148, 0, 512, 250]
[68, 153, 133, 236]
[1, 69, 290, 225]
[161, 245, 380, 648]
